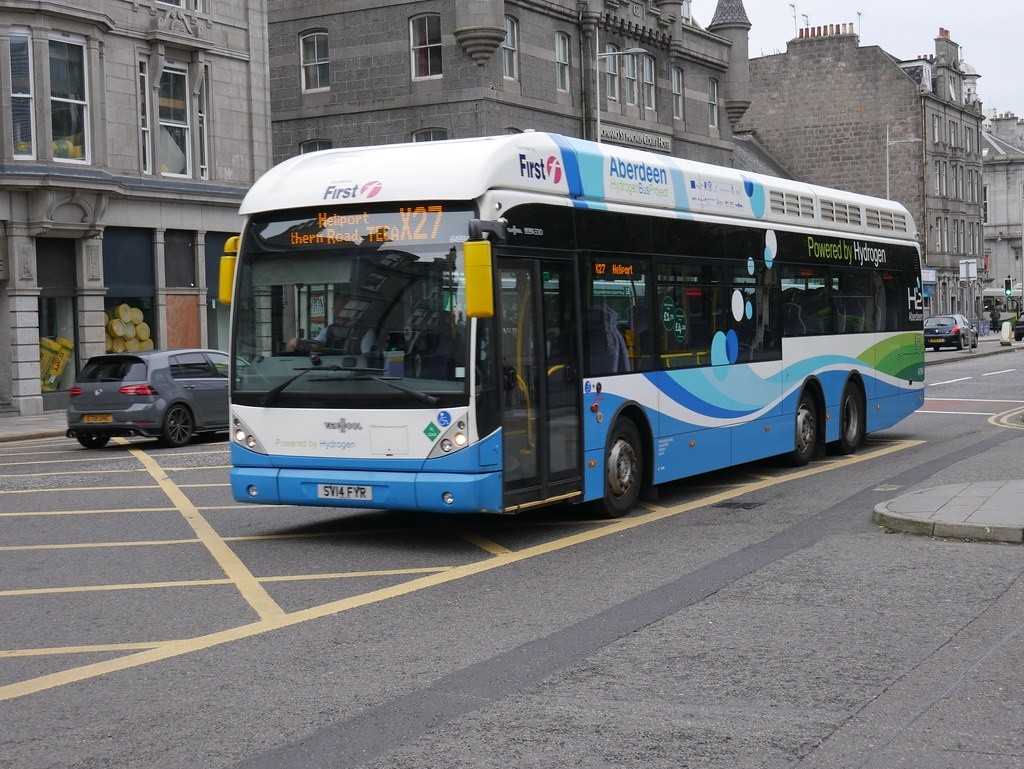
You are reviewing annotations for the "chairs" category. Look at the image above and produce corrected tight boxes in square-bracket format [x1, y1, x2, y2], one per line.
[780, 287, 882, 338]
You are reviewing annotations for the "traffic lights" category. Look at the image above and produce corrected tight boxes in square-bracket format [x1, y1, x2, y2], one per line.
[1004, 278, 1013, 297]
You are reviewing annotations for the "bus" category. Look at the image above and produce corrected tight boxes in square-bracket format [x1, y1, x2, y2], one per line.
[219, 128, 926, 522]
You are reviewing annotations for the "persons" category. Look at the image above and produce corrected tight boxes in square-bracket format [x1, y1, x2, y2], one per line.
[990, 308, 1001, 333]
[290, 295, 376, 355]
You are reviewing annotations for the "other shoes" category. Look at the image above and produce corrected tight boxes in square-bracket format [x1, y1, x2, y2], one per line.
[997, 330, 999, 333]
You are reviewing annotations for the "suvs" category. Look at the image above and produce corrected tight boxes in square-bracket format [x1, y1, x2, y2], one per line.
[1014, 310, 1024, 341]
[923, 313, 979, 351]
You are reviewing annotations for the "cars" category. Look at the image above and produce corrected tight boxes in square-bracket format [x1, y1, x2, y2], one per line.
[66, 347, 270, 449]
